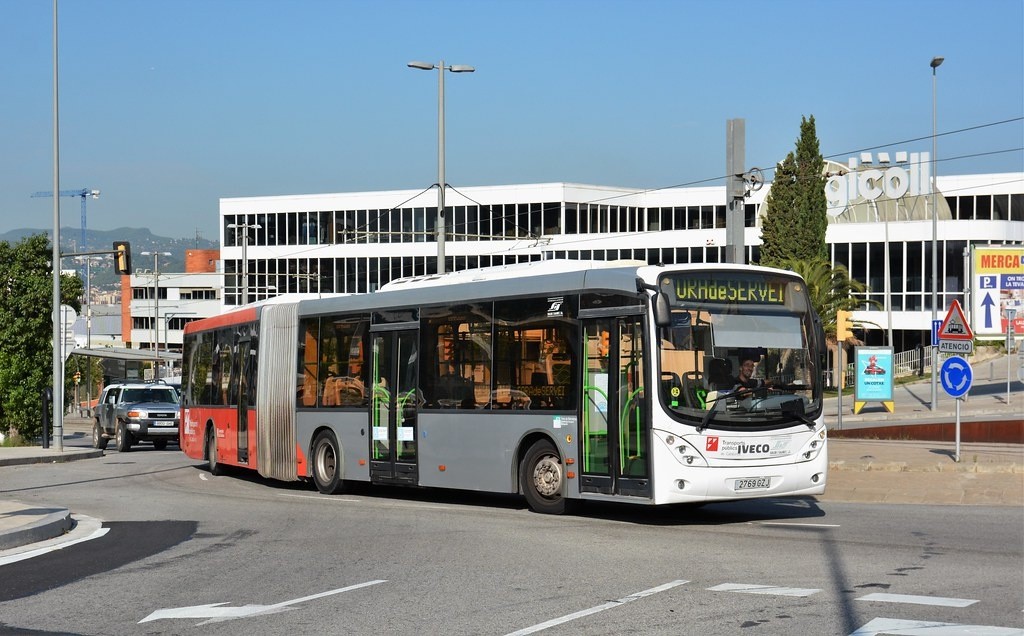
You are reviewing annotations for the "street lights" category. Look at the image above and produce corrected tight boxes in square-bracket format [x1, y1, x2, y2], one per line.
[930, 56, 944, 409]
[227, 223, 262, 276]
[407, 62, 476, 275]
[142, 251, 172, 384]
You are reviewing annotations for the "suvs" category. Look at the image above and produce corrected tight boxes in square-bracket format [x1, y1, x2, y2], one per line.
[92, 378, 179, 452]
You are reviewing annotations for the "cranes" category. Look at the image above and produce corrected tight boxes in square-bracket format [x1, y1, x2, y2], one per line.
[29, 188, 100, 303]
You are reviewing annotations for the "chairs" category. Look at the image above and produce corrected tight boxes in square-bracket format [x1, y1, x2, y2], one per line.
[709, 357, 736, 393]
[660, 371, 706, 409]
[320, 377, 365, 407]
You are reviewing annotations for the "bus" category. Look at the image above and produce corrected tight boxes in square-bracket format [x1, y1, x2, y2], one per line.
[178, 258, 827, 514]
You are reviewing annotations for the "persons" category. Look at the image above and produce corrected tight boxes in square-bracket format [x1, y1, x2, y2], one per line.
[729, 360, 775, 396]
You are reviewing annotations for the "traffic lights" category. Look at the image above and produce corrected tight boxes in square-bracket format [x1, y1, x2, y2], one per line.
[836, 311, 853, 341]
